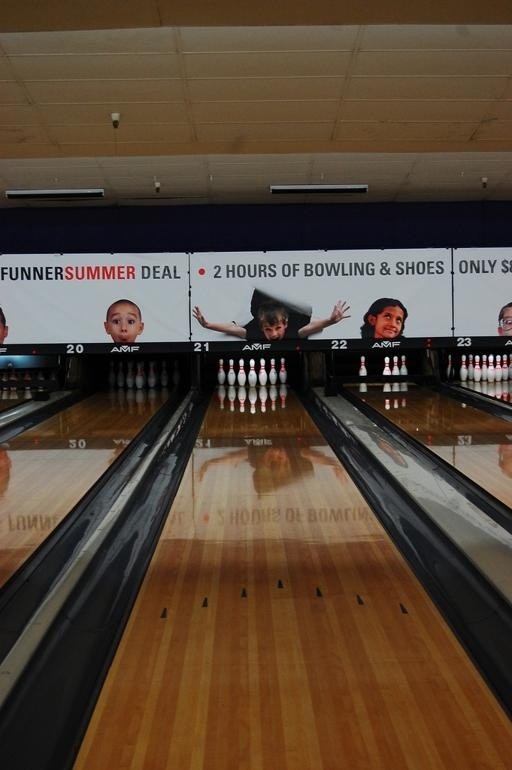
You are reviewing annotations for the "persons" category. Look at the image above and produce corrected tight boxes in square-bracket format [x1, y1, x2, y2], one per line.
[498, 301, 512, 336]
[199, 445, 348, 482]
[0, 308, 8, 345]
[360, 298, 408, 338]
[192, 299, 351, 340]
[357, 424, 409, 467]
[104, 299, 144, 343]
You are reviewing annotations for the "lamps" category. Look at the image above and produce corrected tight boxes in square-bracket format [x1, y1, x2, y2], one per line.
[4, 188, 105, 200]
[269, 183, 369, 195]
